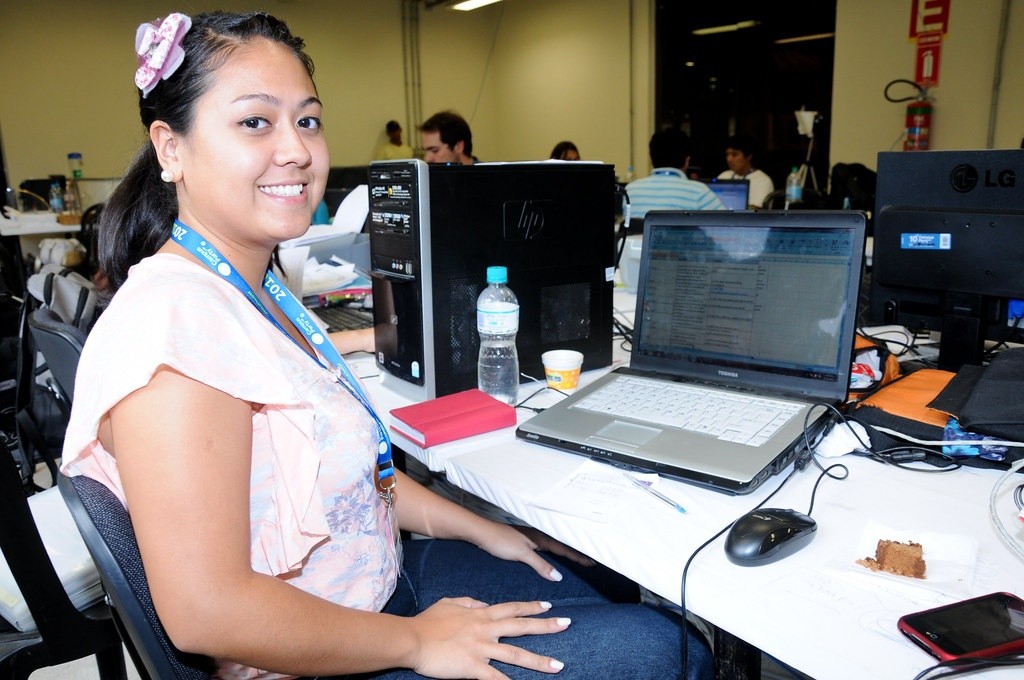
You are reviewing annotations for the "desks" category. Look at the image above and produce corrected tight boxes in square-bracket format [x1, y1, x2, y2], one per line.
[0, 205, 92, 291]
[342, 328, 1024, 679]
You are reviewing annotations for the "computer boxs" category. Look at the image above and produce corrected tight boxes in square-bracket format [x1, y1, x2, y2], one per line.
[366, 158, 618, 401]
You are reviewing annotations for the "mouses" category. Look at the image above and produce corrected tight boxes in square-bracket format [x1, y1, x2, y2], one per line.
[723, 508, 818, 568]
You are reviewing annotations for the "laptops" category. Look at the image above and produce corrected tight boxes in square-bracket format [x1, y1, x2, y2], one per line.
[523, 207, 869, 496]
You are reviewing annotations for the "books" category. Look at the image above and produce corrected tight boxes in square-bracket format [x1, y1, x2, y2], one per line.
[389, 387, 517, 450]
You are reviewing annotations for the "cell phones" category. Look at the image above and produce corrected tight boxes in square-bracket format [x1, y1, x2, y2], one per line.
[897, 591, 1024, 669]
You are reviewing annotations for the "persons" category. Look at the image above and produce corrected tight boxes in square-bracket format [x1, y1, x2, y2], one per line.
[374, 120, 414, 160]
[415, 112, 482, 165]
[59, 11, 715, 680]
[714, 135, 774, 210]
[623, 125, 728, 221]
[550, 142, 580, 161]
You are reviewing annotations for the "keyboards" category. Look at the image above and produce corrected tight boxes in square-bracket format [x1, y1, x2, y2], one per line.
[311, 303, 376, 334]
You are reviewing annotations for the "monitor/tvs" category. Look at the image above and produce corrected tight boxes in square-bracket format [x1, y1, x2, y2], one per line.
[698, 179, 750, 211]
[873, 150, 1024, 373]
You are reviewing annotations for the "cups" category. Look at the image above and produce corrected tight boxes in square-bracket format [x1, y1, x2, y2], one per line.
[542, 349, 583, 399]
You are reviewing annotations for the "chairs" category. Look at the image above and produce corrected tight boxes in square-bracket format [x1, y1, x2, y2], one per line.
[0, 203, 221, 680]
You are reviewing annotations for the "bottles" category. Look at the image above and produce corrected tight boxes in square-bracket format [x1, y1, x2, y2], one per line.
[786, 168, 801, 210]
[626, 167, 634, 183]
[50, 182, 77, 213]
[68, 153, 82, 179]
[477, 266, 520, 408]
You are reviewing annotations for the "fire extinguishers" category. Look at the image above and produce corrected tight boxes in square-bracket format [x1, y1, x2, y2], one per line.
[885, 79, 936, 150]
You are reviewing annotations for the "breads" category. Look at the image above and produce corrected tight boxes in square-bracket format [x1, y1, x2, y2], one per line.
[857, 539, 926, 579]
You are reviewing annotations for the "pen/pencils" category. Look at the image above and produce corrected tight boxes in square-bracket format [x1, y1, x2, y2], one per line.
[622, 471, 687, 514]
